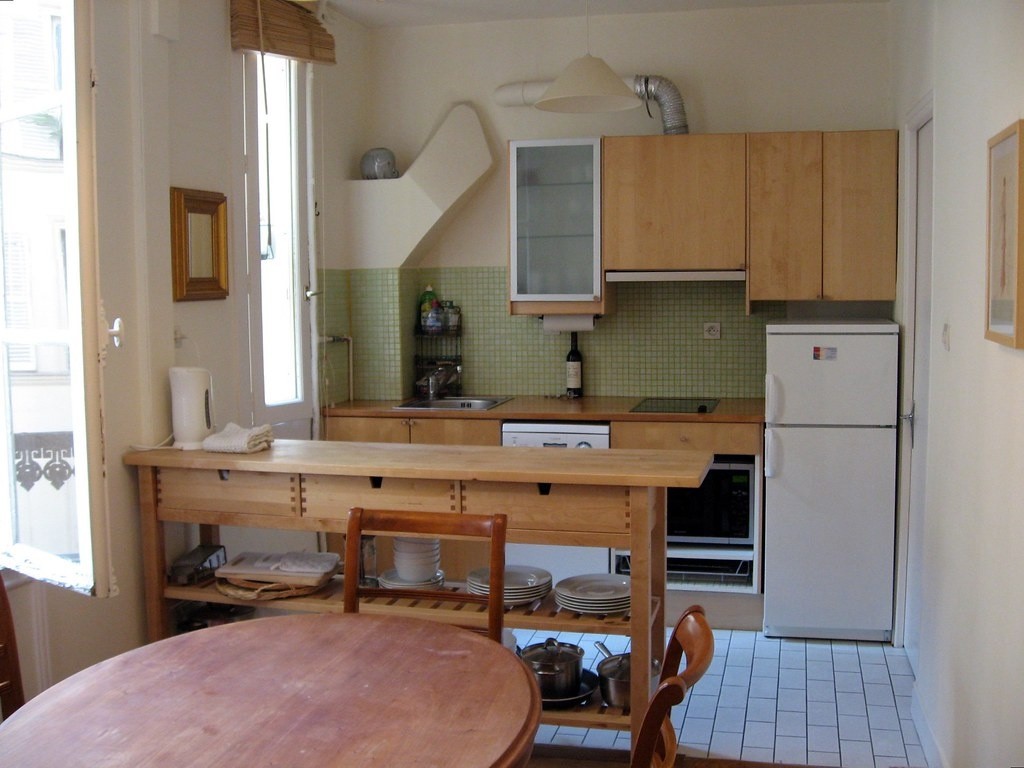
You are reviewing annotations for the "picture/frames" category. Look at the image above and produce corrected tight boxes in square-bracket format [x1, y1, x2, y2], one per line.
[987, 119, 1024, 350]
[168, 186, 231, 301]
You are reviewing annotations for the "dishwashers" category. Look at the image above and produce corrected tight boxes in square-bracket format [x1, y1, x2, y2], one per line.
[500, 423, 609, 588]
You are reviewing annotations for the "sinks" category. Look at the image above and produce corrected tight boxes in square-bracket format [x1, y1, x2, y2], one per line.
[391, 395, 516, 412]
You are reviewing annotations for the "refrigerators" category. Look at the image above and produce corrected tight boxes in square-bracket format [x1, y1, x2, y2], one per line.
[764, 317, 899, 643]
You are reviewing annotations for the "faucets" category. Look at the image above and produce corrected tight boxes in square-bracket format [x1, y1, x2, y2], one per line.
[428, 365, 464, 398]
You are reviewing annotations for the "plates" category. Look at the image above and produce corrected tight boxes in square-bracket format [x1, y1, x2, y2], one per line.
[555, 574, 632, 614]
[378, 569, 445, 594]
[466, 565, 553, 607]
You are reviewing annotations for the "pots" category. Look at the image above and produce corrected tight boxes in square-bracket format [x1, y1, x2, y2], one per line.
[517, 638, 632, 708]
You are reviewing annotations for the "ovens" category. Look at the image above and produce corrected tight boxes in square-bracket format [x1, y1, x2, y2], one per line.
[615, 456, 757, 591]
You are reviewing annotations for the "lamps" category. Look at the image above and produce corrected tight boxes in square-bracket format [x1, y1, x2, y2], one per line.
[535, 0, 642, 113]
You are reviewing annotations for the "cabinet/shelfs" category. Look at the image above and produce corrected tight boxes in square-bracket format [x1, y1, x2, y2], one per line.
[610, 420, 761, 456]
[750, 129, 898, 301]
[604, 132, 747, 271]
[415, 315, 465, 395]
[324, 417, 502, 445]
[508, 135, 603, 316]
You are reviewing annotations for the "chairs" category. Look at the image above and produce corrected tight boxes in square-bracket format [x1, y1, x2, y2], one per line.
[630, 606, 714, 768]
[342, 506, 507, 646]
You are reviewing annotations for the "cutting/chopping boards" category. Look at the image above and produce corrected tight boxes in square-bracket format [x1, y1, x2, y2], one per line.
[216, 550, 340, 587]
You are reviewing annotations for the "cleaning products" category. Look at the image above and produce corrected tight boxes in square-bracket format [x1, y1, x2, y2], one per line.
[419, 284, 444, 334]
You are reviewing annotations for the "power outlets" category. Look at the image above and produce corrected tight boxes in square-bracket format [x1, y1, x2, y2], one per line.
[174, 325, 183, 349]
[705, 322, 720, 337]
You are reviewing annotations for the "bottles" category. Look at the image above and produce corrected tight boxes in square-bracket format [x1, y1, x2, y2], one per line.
[566, 331, 583, 396]
[342, 534, 379, 592]
[421, 285, 459, 335]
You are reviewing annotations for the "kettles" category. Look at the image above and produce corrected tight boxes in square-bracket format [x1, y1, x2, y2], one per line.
[170, 366, 216, 453]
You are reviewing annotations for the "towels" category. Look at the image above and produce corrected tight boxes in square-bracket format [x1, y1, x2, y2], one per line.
[202, 422, 275, 453]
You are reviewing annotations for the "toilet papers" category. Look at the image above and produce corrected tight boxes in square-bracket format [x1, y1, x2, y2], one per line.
[542, 314, 597, 331]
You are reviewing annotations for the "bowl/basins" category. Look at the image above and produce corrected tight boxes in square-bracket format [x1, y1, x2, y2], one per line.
[391, 536, 442, 582]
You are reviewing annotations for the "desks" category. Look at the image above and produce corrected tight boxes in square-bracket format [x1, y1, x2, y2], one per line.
[0, 612, 544, 767]
[120, 431, 717, 767]
